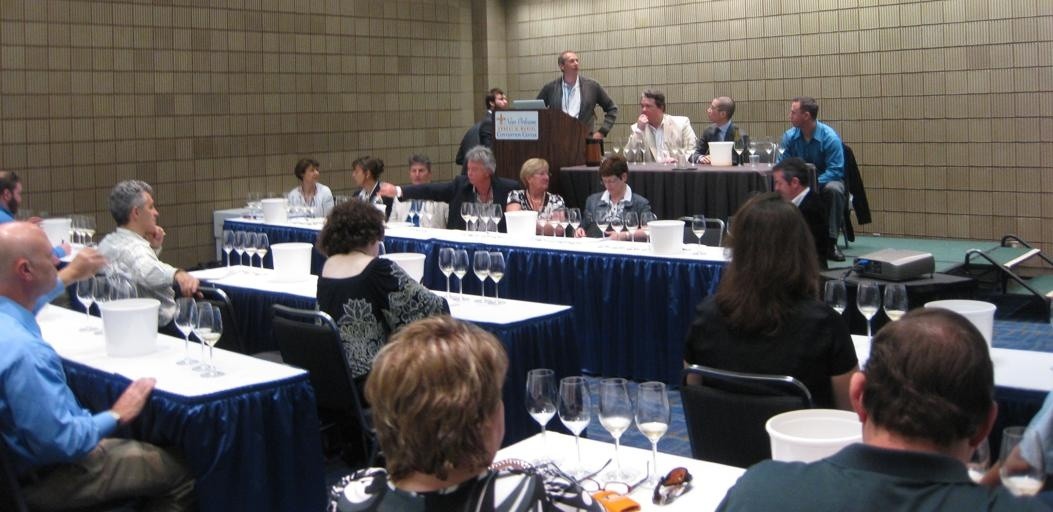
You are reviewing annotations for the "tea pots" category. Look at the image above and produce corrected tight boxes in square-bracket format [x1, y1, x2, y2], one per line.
[582, 136, 604, 167]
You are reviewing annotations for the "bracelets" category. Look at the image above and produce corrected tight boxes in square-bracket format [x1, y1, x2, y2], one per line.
[625, 231, 630, 241]
[108, 411, 123, 423]
[174, 268, 188, 281]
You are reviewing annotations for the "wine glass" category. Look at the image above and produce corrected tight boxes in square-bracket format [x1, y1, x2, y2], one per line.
[611, 135, 649, 167]
[458, 200, 504, 239]
[245, 190, 386, 225]
[435, 247, 507, 306]
[219, 229, 270, 275]
[524, 364, 671, 487]
[73, 271, 139, 335]
[821, 278, 908, 354]
[403, 197, 436, 229]
[171, 296, 227, 381]
[66, 213, 98, 248]
[595, 210, 655, 254]
[536, 206, 583, 240]
[732, 134, 786, 168]
[658, 136, 698, 167]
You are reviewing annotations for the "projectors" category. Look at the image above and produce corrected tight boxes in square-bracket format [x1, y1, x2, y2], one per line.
[854, 247, 935, 280]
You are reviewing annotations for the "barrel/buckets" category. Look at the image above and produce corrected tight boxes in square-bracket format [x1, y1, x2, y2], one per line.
[764, 407, 864, 464]
[923, 298, 997, 360]
[377, 252, 427, 287]
[503, 209, 539, 240]
[38, 217, 72, 249]
[270, 242, 313, 284]
[97, 297, 162, 360]
[647, 219, 686, 256]
[261, 198, 289, 225]
[707, 141, 734, 167]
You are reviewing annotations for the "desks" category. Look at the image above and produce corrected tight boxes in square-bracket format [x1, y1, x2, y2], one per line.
[0, 160, 1053, 512]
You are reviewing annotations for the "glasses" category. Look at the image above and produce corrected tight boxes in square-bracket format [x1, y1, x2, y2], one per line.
[577, 458, 649, 495]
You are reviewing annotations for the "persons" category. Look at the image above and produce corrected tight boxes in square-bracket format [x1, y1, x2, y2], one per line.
[978, 386, 1053, 488]
[379, 146, 523, 233]
[575, 155, 654, 241]
[772, 157, 828, 271]
[323, 316, 602, 512]
[682, 193, 860, 411]
[687, 96, 752, 166]
[0, 221, 197, 512]
[508, 159, 576, 239]
[98, 178, 203, 340]
[712, 308, 1053, 512]
[314, 198, 449, 404]
[534, 51, 617, 140]
[387, 156, 450, 229]
[290, 159, 333, 218]
[0, 172, 70, 267]
[454, 120, 480, 178]
[772, 96, 844, 261]
[622, 89, 698, 163]
[346, 155, 392, 220]
[478, 88, 508, 146]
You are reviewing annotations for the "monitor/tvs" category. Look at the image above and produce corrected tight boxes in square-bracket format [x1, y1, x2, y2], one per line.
[513, 99, 549, 109]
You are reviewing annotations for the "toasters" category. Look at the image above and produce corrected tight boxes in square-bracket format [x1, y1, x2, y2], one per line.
[689, 214, 708, 255]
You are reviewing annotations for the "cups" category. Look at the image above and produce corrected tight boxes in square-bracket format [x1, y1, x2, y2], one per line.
[966, 436, 992, 483]
[1000, 424, 1047, 497]
